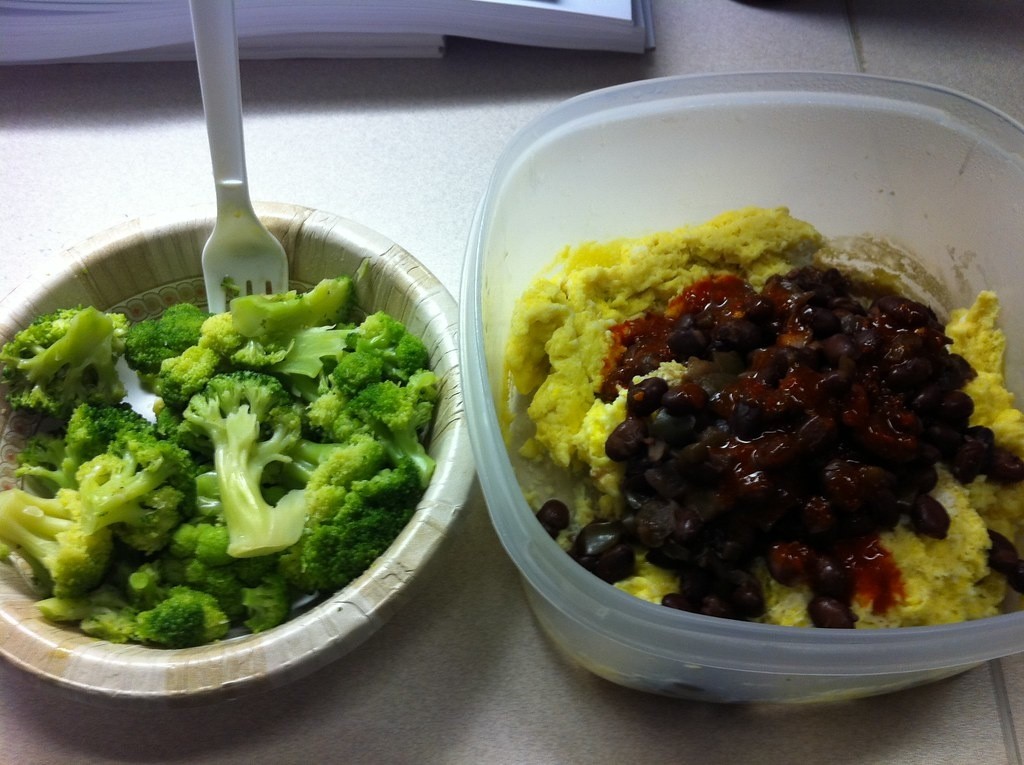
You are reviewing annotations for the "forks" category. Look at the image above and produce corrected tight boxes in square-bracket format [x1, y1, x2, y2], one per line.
[189, 2, 286, 314]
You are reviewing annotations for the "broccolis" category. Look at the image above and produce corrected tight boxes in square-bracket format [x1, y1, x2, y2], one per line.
[0, 273, 440, 649]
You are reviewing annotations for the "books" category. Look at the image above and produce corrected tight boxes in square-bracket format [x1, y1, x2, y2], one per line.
[0, 0, 657, 66]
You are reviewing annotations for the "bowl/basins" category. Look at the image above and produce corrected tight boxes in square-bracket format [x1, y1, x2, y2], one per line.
[0, 203, 476, 699]
[460, 69, 1024, 708]
